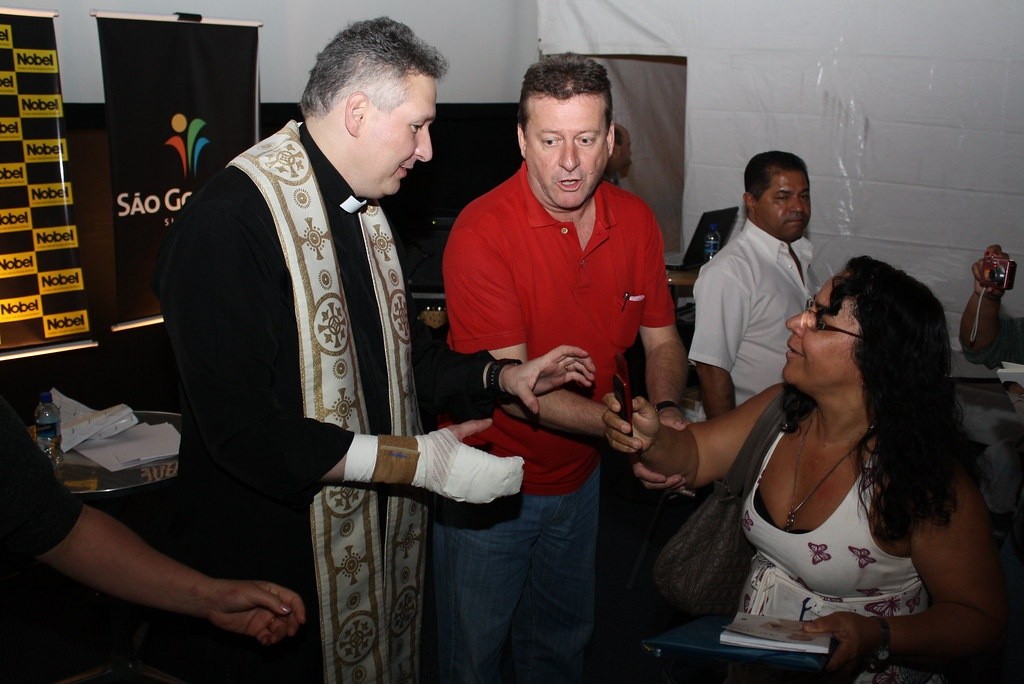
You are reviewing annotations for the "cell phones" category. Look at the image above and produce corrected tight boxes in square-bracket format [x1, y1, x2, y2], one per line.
[613, 375, 633, 438]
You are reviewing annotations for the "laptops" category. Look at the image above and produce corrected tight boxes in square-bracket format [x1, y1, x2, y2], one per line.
[664, 206, 740, 270]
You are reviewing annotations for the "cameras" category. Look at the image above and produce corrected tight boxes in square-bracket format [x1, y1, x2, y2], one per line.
[980, 257, 1017, 291]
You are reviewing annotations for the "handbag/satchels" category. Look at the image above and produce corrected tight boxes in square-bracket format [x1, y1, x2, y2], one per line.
[651, 385, 799, 616]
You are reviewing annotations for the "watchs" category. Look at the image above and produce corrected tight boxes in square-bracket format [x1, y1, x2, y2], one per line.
[870, 617, 890, 660]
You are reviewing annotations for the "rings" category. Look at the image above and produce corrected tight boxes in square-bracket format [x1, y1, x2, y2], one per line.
[268, 618, 275, 626]
[565, 366, 568, 370]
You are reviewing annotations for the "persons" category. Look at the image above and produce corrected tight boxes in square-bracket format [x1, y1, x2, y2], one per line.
[433, 52, 688, 684]
[603, 256, 1008, 684]
[0, 396, 306, 646]
[688, 151, 821, 421]
[158, 17, 597, 684]
[603, 123, 632, 184]
[959, 244, 1024, 370]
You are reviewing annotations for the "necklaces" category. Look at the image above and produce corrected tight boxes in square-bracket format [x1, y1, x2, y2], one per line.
[785, 407, 855, 532]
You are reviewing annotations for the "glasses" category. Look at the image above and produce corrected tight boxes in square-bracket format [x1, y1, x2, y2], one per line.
[805, 298, 863, 340]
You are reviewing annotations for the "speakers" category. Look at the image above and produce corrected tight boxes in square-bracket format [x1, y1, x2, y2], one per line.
[401, 217, 457, 294]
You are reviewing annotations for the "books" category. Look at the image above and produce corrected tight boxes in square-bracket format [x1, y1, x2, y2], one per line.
[997, 361, 1024, 422]
[719, 612, 832, 654]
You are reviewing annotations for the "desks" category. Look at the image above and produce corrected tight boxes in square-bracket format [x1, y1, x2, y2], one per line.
[22, 410, 186, 684]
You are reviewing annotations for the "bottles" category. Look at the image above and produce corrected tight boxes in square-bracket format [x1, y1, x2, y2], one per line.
[33, 391, 65, 476]
[704, 223, 721, 264]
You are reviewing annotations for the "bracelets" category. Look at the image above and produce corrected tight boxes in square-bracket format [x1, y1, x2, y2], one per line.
[654, 401, 678, 412]
[486, 358, 522, 394]
